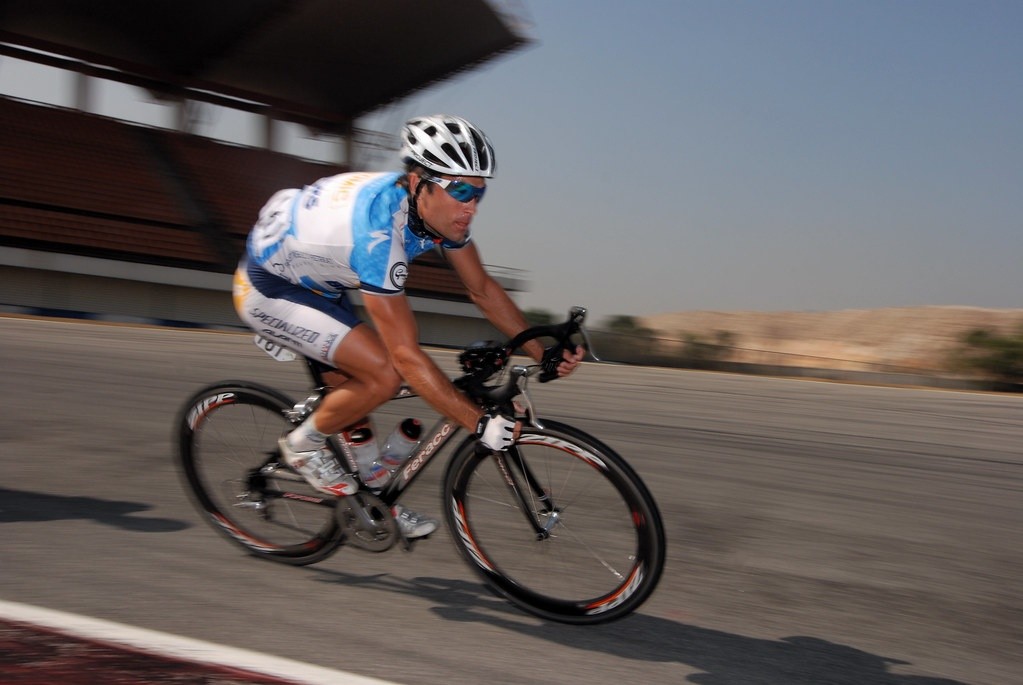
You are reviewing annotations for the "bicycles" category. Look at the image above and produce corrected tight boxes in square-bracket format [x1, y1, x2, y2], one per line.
[168, 302, 666, 628]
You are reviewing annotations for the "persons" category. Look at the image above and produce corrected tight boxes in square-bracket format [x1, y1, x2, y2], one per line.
[232, 114, 587, 539]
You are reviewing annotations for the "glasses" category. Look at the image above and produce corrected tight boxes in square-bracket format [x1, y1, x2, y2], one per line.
[420, 170, 486, 204]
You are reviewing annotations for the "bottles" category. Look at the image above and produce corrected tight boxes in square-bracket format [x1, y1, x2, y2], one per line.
[350, 429, 388, 492]
[370, 417, 423, 484]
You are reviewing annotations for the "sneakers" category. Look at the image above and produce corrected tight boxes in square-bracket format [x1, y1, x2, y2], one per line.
[389, 503, 437, 539]
[277, 431, 359, 496]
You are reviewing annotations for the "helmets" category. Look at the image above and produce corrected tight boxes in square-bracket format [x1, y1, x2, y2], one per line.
[400, 113, 496, 179]
